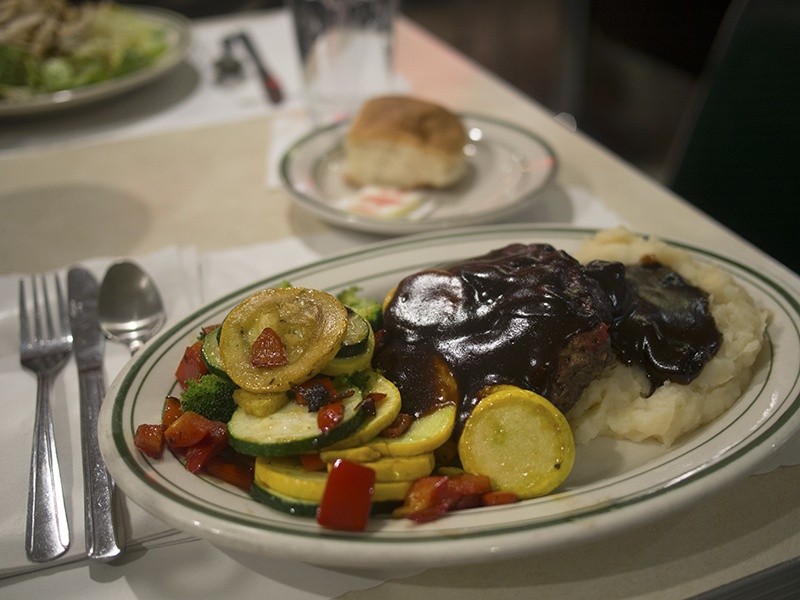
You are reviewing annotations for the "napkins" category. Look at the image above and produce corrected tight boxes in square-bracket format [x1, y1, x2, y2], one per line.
[37, 3, 304, 156]
[0, 242, 322, 580]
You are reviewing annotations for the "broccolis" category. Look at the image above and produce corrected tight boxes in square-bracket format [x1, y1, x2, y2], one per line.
[0, 38, 38, 89]
[179, 374, 239, 425]
[336, 286, 386, 329]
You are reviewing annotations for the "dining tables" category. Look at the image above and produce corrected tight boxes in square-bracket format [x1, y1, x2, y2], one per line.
[0, 1, 798, 599]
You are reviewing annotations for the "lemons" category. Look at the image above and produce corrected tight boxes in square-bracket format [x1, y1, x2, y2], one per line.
[254, 370, 575, 518]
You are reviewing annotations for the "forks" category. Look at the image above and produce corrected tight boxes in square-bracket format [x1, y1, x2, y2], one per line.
[19, 271, 75, 563]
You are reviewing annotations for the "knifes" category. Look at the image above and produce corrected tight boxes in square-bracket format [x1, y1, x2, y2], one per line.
[67, 264, 123, 565]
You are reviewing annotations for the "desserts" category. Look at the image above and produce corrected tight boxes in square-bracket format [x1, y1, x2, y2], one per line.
[340, 92, 472, 193]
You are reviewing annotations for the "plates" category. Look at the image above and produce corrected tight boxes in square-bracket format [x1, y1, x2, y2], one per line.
[0, 7, 189, 114]
[280, 111, 562, 237]
[95, 221, 800, 566]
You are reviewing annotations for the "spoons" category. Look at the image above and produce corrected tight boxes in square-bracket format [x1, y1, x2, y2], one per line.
[98, 260, 167, 356]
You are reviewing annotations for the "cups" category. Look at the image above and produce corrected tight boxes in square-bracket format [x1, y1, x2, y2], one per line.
[292, 0, 396, 124]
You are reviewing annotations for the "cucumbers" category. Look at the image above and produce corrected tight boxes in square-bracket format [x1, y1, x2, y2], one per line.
[203, 305, 371, 457]
